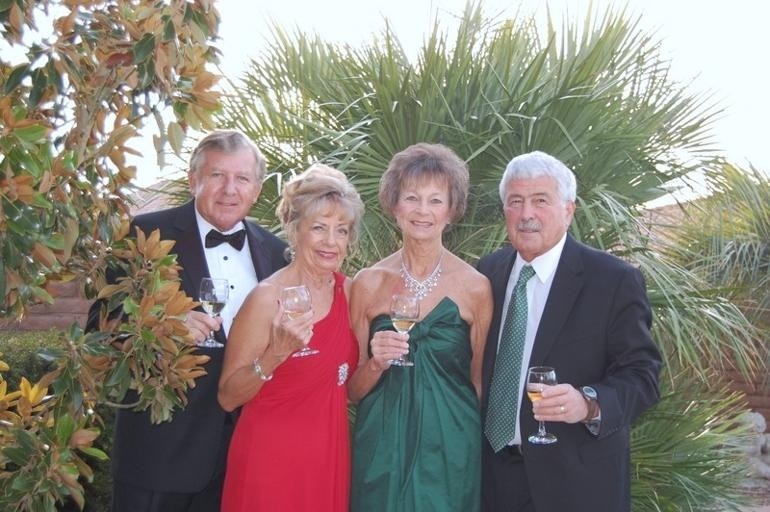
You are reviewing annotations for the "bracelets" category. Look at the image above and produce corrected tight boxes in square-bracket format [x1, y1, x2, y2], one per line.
[254, 357, 273, 382]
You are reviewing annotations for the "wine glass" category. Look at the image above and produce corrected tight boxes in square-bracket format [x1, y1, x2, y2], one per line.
[386, 294, 421, 368]
[526, 365, 559, 446]
[195, 276, 231, 349]
[278, 284, 320, 358]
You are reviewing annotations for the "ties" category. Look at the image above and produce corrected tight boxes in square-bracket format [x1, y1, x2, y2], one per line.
[484, 266, 535, 455]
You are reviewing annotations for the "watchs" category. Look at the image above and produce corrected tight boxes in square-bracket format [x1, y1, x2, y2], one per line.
[576, 385, 598, 422]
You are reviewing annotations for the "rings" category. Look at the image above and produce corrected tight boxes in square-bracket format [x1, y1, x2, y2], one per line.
[306, 326, 312, 335]
[560, 405, 564, 413]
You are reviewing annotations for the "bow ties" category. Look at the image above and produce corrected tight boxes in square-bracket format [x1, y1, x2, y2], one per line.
[204, 228, 247, 251]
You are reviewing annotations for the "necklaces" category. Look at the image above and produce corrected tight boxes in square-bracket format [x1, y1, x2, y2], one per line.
[398, 247, 446, 299]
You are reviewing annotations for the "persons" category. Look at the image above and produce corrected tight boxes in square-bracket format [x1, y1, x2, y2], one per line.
[345, 141, 495, 512]
[83, 128, 293, 512]
[217, 161, 361, 512]
[473, 150, 662, 512]
